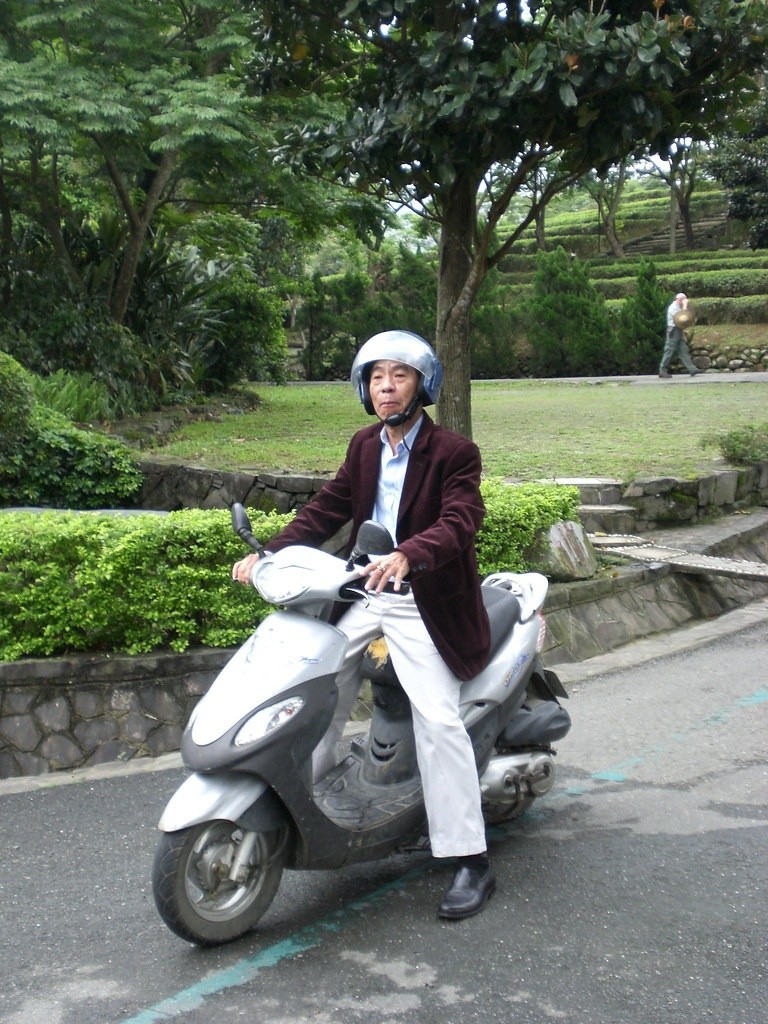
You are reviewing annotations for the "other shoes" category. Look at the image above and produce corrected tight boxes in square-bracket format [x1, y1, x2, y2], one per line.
[659, 373, 672, 378]
[691, 368, 704, 377]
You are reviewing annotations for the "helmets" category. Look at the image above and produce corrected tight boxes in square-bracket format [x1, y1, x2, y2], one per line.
[349, 330, 443, 415]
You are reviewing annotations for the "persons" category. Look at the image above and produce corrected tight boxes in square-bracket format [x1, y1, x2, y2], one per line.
[658, 293, 703, 378]
[229, 330, 497, 920]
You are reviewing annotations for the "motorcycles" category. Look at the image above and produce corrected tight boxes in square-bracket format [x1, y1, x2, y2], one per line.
[150, 502, 575, 949]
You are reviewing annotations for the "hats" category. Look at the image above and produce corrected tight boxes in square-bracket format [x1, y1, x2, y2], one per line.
[674, 310, 694, 330]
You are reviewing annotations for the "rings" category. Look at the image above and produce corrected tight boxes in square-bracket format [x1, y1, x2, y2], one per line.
[376, 565, 386, 573]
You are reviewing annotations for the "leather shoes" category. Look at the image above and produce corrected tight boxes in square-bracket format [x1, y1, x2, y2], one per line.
[437, 856, 496, 919]
